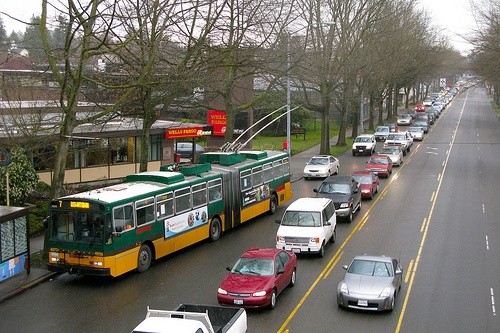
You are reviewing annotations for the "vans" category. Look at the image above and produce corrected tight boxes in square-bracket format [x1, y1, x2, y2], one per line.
[275, 198, 336, 258]
[312, 175, 361, 223]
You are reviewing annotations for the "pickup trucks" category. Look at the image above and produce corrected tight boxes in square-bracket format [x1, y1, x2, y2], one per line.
[131, 303, 247, 333]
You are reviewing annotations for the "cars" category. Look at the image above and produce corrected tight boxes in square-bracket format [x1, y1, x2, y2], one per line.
[303, 155, 339, 180]
[377, 146, 404, 167]
[373, 124, 398, 142]
[383, 132, 414, 156]
[174, 143, 204, 158]
[337, 255, 404, 312]
[352, 135, 377, 155]
[353, 172, 380, 200]
[217, 248, 297, 309]
[365, 155, 393, 178]
[396, 89, 457, 141]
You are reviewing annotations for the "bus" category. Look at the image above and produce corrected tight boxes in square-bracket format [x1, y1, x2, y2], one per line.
[43, 151, 293, 277]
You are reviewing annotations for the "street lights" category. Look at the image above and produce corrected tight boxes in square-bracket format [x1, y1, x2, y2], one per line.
[287, 21, 335, 155]
